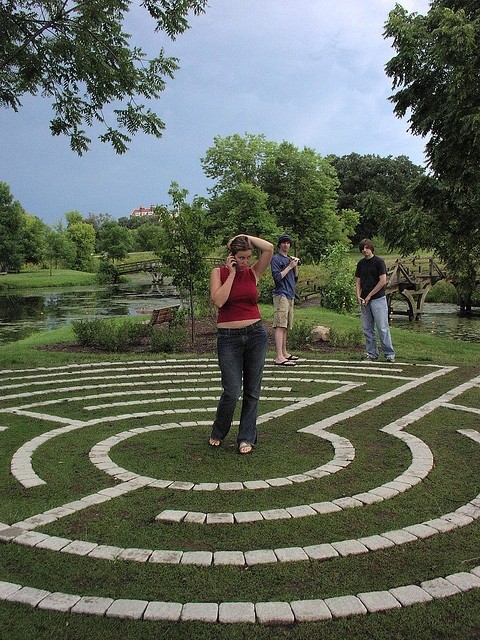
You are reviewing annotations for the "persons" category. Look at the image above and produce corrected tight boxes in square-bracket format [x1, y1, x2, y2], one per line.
[271, 234, 300, 366]
[209, 234, 273, 453]
[355, 239, 396, 361]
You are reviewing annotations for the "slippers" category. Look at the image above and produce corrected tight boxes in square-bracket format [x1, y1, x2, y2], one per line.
[274, 360, 296, 366]
[285, 354, 298, 360]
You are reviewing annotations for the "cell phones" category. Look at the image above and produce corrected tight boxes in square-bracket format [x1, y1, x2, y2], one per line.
[230, 254, 237, 267]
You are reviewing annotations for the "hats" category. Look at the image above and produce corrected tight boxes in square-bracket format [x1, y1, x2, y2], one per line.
[359, 238, 374, 251]
[277, 235, 292, 248]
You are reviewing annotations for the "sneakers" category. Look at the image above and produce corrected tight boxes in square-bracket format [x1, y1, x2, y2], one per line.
[362, 355, 379, 362]
[388, 359, 395, 362]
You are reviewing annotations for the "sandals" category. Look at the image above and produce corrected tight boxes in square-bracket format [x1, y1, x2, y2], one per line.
[209, 436, 222, 446]
[239, 440, 253, 454]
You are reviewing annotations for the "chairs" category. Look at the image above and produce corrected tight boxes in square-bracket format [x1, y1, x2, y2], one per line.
[148, 304, 180, 327]
